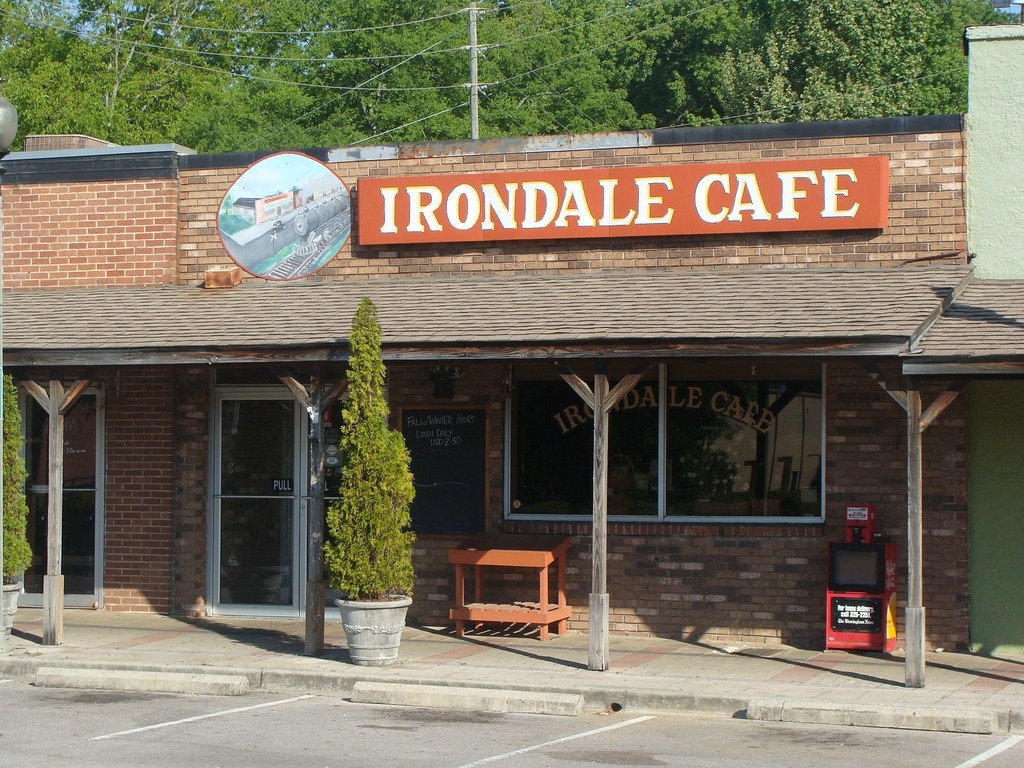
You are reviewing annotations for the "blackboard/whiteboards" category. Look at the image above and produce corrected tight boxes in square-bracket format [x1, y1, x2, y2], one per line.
[396, 403, 492, 540]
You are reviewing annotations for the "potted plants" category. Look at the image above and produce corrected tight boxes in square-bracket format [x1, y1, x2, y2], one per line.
[320, 298, 417, 666]
[3, 374, 33, 654]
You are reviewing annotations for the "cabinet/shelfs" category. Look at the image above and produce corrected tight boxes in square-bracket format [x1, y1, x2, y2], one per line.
[448, 532, 573, 641]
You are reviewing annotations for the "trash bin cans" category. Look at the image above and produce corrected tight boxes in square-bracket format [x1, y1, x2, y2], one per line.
[824, 505, 897, 654]
